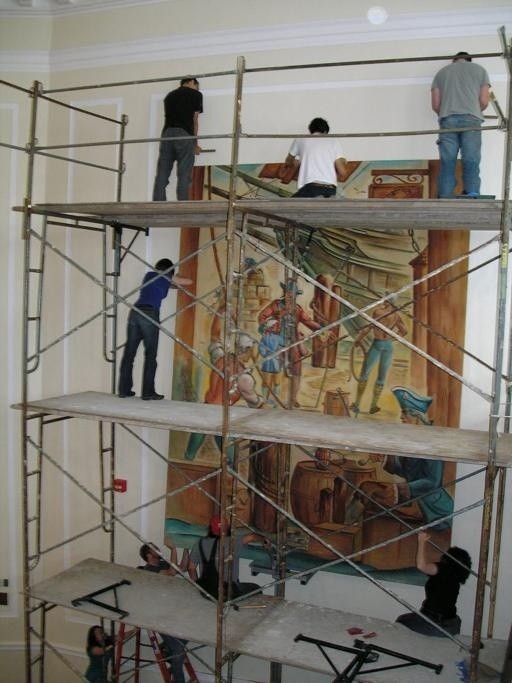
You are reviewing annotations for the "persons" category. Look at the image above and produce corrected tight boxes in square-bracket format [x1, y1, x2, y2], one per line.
[187, 298, 265, 473]
[260, 277, 338, 410]
[118, 258, 193, 401]
[258, 317, 290, 409]
[138, 538, 189, 577]
[188, 515, 271, 602]
[287, 117, 350, 198]
[395, 531, 472, 637]
[350, 291, 408, 413]
[85, 625, 144, 682]
[356, 387, 454, 530]
[159, 632, 189, 683]
[431, 50, 492, 199]
[154, 77, 202, 202]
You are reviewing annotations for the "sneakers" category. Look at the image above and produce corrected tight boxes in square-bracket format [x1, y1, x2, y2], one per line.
[143, 393, 164, 400]
[119, 391, 135, 398]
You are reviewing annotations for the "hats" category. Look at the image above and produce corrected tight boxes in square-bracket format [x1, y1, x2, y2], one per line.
[391, 386, 432, 413]
[236, 335, 253, 348]
[280, 278, 304, 294]
[209, 515, 228, 536]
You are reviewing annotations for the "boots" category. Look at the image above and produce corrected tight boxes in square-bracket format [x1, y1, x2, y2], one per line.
[273, 384, 288, 407]
[350, 379, 367, 409]
[370, 384, 384, 413]
[262, 386, 273, 404]
[285, 374, 299, 409]
